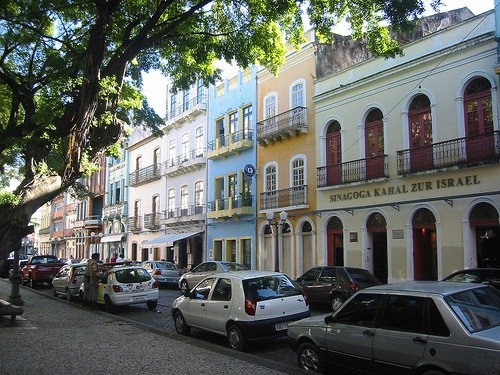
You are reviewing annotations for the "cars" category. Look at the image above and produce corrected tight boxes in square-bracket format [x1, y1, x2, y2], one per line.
[171, 270, 311, 351]
[178, 261, 251, 295]
[20, 255, 179, 313]
[287, 268, 500, 374]
[294, 266, 382, 312]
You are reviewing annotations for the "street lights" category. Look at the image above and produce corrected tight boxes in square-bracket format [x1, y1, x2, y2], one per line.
[91, 231, 103, 252]
[51, 236, 62, 257]
[266, 209, 287, 272]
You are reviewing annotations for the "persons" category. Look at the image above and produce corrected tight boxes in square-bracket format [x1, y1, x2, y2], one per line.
[69, 255, 74, 259]
[111, 254, 129, 262]
[84, 253, 108, 310]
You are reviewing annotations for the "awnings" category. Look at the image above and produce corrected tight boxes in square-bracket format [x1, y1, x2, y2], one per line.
[139, 231, 201, 248]
[100, 235, 124, 242]
